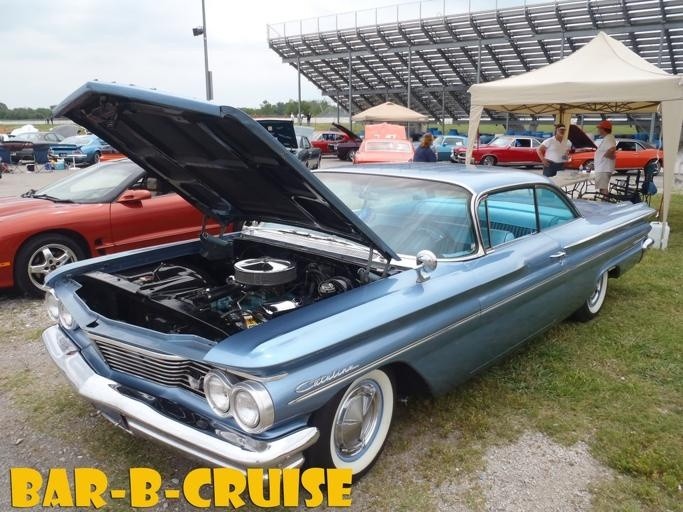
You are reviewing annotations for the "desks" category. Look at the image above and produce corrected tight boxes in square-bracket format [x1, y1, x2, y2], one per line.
[552, 172, 637, 198]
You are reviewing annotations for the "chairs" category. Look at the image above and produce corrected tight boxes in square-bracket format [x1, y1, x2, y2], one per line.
[607, 171, 642, 204]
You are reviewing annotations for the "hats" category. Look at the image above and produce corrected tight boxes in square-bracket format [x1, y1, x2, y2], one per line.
[596, 121, 612, 128]
[557, 124, 566, 130]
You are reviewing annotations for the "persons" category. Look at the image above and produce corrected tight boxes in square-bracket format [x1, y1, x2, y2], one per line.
[594, 120, 616, 201]
[290, 112, 311, 126]
[537, 123, 572, 177]
[414, 133, 437, 161]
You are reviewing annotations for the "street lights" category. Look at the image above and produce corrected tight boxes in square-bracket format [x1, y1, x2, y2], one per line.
[192, 25, 213, 101]
